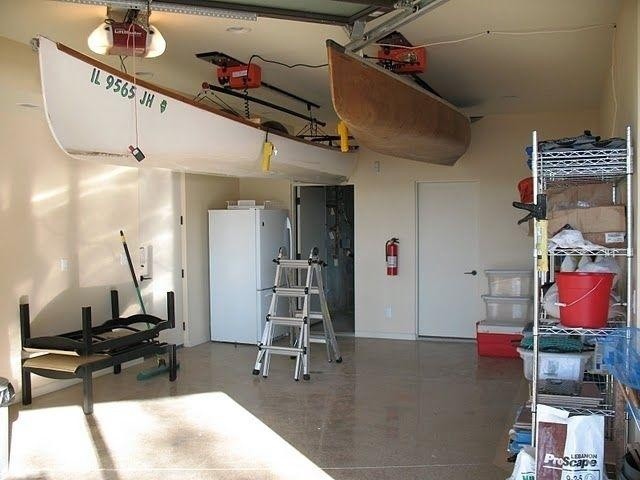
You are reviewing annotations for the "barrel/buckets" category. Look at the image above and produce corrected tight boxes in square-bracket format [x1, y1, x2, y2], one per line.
[553, 270, 618, 328]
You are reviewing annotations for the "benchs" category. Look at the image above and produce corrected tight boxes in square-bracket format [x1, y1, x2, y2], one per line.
[21, 342, 176, 414]
[19, 290, 175, 357]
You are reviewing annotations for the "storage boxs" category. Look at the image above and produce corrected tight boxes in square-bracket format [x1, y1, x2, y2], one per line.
[485, 271, 534, 298]
[544, 182, 613, 210]
[476, 321, 525, 358]
[517, 346, 594, 383]
[482, 296, 532, 325]
[549, 205, 627, 248]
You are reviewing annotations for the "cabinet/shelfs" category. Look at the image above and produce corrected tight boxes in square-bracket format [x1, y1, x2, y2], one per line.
[531, 127, 633, 454]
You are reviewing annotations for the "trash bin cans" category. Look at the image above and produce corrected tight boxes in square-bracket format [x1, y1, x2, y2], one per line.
[0, 377, 16, 473]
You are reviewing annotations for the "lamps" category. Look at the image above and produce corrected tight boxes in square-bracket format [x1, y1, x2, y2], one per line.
[88, 21, 167, 58]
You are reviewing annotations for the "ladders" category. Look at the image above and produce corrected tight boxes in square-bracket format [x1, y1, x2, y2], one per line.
[252, 247, 343, 380]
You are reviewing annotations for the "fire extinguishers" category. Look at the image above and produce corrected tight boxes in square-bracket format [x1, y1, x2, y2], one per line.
[385, 238, 400, 275]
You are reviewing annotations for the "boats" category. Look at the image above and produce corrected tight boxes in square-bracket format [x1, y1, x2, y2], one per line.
[36, 37, 360, 187]
